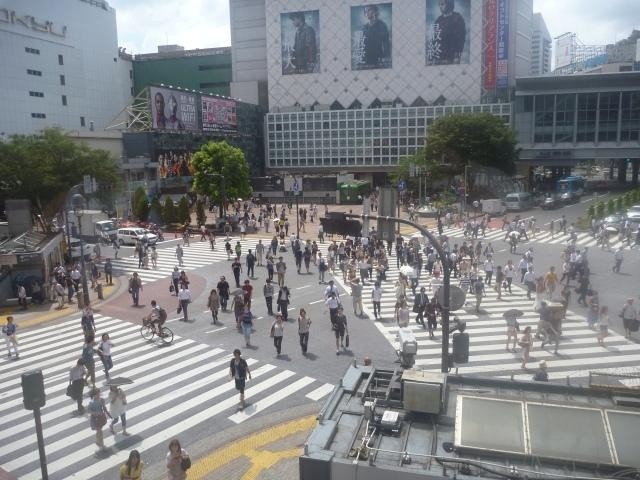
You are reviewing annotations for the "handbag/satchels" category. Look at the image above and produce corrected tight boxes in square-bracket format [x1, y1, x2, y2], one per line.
[181, 455, 191, 471]
[412, 305, 419, 313]
[169, 284, 175, 292]
[270, 322, 276, 338]
[345, 334, 349, 347]
[177, 306, 182, 314]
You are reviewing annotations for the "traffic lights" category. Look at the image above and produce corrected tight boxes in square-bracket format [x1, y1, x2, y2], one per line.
[319, 217, 363, 237]
[22, 369, 46, 411]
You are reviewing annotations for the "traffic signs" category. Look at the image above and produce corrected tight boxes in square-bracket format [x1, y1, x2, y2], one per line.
[293, 182, 299, 196]
[398, 180, 406, 191]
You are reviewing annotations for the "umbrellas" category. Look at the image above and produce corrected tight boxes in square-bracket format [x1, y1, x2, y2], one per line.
[105, 377, 134, 386]
[230, 288, 247, 295]
[503, 309, 524, 317]
[400, 265, 415, 277]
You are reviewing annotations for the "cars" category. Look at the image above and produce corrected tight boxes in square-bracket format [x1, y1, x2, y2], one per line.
[604, 214, 623, 232]
[626, 205, 640, 219]
[540, 198, 560, 209]
[560, 192, 580, 204]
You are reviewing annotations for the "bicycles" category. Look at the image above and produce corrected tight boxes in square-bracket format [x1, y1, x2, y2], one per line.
[141, 317, 173, 343]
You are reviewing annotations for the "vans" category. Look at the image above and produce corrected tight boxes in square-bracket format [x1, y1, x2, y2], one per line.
[506, 192, 531, 211]
[117, 228, 157, 245]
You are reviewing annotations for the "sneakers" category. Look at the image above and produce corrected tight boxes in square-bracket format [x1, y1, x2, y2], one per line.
[122, 431, 130, 436]
[336, 350, 339, 354]
[109, 424, 115, 433]
[238, 405, 243, 409]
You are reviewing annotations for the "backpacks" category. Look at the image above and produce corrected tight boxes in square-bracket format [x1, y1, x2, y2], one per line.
[154, 307, 167, 321]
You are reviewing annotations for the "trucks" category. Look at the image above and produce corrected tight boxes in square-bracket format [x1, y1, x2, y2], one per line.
[482, 199, 507, 216]
[67, 210, 117, 241]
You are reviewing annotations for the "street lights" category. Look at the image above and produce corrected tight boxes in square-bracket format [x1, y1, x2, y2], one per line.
[72, 194, 86, 277]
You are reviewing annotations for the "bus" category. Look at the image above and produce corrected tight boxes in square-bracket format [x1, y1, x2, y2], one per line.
[556, 176, 584, 198]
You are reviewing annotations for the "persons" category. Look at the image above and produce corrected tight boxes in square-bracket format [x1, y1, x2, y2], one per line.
[224, 242, 231, 260]
[178, 284, 192, 321]
[351, 279, 364, 316]
[109, 386, 130, 437]
[217, 275, 229, 310]
[278, 286, 290, 319]
[97, 333, 115, 384]
[213, 205, 217, 217]
[155, 92, 167, 129]
[128, 272, 143, 306]
[361, 5, 388, 69]
[157, 150, 198, 178]
[105, 258, 112, 285]
[148, 300, 166, 335]
[200, 226, 207, 241]
[372, 282, 382, 319]
[290, 13, 316, 73]
[324, 293, 341, 325]
[230, 349, 251, 409]
[53, 262, 65, 283]
[394, 203, 640, 381]
[238, 307, 256, 346]
[82, 335, 102, 390]
[233, 194, 393, 284]
[120, 450, 143, 480]
[88, 389, 112, 448]
[64, 275, 77, 302]
[179, 271, 189, 289]
[69, 358, 86, 415]
[208, 201, 212, 214]
[270, 315, 283, 358]
[16, 282, 27, 311]
[224, 223, 232, 240]
[70, 267, 82, 291]
[171, 267, 181, 296]
[264, 279, 275, 315]
[298, 308, 311, 357]
[208, 289, 220, 324]
[165, 439, 191, 480]
[433, 0, 465, 65]
[112, 241, 119, 258]
[2, 316, 20, 359]
[134, 223, 163, 269]
[208, 230, 216, 249]
[51, 281, 65, 310]
[325, 282, 339, 298]
[81, 307, 96, 341]
[175, 244, 184, 266]
[332, 307, 347, 355]
